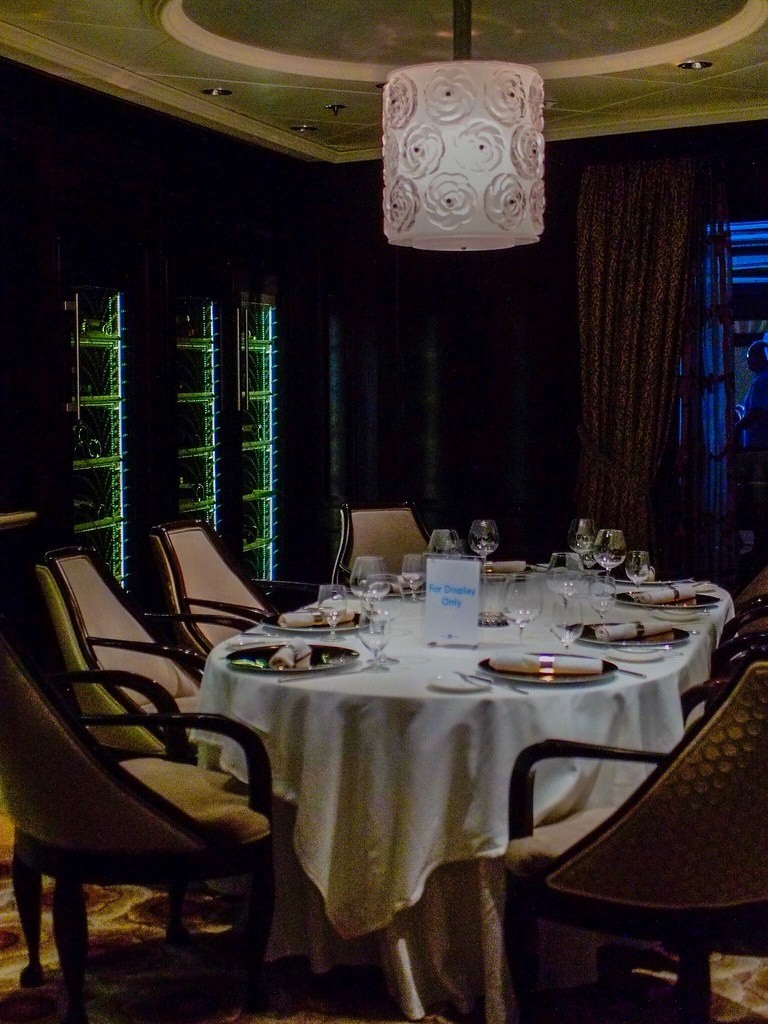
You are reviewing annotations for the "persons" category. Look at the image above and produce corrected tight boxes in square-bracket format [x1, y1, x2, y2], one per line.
[719, 340, 768, 460]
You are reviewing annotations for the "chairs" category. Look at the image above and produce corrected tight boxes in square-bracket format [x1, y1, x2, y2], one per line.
[37, 541, 257, 757]
[330, 496, 450, 591]
[500, 566, 768, 1024]
[146, 517, 328, 661]
[0, 554, 276, 1024]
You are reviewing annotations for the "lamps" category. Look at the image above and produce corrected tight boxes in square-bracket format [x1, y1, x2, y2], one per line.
[379, 0, 548, 252]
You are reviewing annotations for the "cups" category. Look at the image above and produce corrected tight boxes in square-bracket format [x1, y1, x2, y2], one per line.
[476, 573, 508, 626]
[428, 529, 462, 558]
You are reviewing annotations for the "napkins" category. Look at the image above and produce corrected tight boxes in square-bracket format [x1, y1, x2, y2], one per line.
[269, 637, 315, 667]
[486, 560, 527, 574]
[595, 617, 674, 641]
[627, 581, 698, 604]
[489, 650, 606, 674]
[278, 602, 359, 628]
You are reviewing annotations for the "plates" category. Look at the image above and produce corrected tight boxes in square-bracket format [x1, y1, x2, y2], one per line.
[615, 591, 723, 608]
[351, 585, 425, 597]
[607, 647, 665, 661]
[598, 569, 695, 584]
[224, 644, 360, 672]
[265, 622, 370, 632]
[576, 623, 689, 646]
[476, 652, 618, 684]
[425, 673, 493, 691]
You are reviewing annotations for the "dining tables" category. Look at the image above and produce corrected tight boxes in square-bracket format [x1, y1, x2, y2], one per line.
[187, 571, 740, 1024]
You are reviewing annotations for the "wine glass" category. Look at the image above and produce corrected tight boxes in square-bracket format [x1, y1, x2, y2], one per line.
[350, 555, 426, 673]
[501, 519, 651, 649]
[318, 585, 347, 642]
[469, 520, 498, 568]
